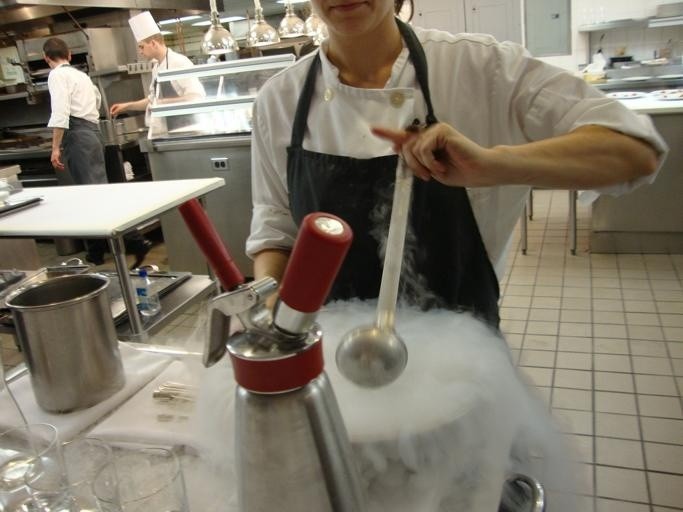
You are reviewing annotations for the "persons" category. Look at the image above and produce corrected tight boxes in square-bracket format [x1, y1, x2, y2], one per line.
[109, 34, 207, 131]
[245, 0, 670, 335]
[43, 37, 153, 269]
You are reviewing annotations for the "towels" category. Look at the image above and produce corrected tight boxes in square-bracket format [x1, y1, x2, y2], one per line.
[0, 340, 176, 448]
[86, 360, 222, 456]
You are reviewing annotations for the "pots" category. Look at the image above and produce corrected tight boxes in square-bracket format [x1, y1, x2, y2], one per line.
[609, 57, 632, 67]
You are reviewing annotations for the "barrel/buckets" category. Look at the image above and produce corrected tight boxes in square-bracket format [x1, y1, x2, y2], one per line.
[3, 274, 125, 413]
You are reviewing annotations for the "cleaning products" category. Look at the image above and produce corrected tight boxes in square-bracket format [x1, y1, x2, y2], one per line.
[136, 268, 161, 316]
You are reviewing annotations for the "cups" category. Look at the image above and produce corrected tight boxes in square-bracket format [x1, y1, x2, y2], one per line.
[0, 422, 189, 511]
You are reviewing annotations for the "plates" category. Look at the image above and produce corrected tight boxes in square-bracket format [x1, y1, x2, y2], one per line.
[603, 74, 682, 103]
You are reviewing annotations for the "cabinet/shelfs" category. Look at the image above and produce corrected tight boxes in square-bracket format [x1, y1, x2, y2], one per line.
[578, 13, 683, 88]
[0, 1, 133, 187]
[137, 52, 297, 152]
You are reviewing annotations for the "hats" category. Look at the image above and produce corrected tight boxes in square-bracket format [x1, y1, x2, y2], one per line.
[128, 10, 161, 43]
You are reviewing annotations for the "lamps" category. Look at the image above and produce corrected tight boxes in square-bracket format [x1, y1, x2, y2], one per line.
[200, 0, 325, 55]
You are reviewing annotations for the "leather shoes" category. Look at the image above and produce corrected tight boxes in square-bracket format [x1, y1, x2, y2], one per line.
[133, 246, 150, 270]
[86, 254, 103, 265]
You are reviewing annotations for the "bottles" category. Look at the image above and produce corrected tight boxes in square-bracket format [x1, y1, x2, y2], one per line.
[130, 269, 165, 318]
